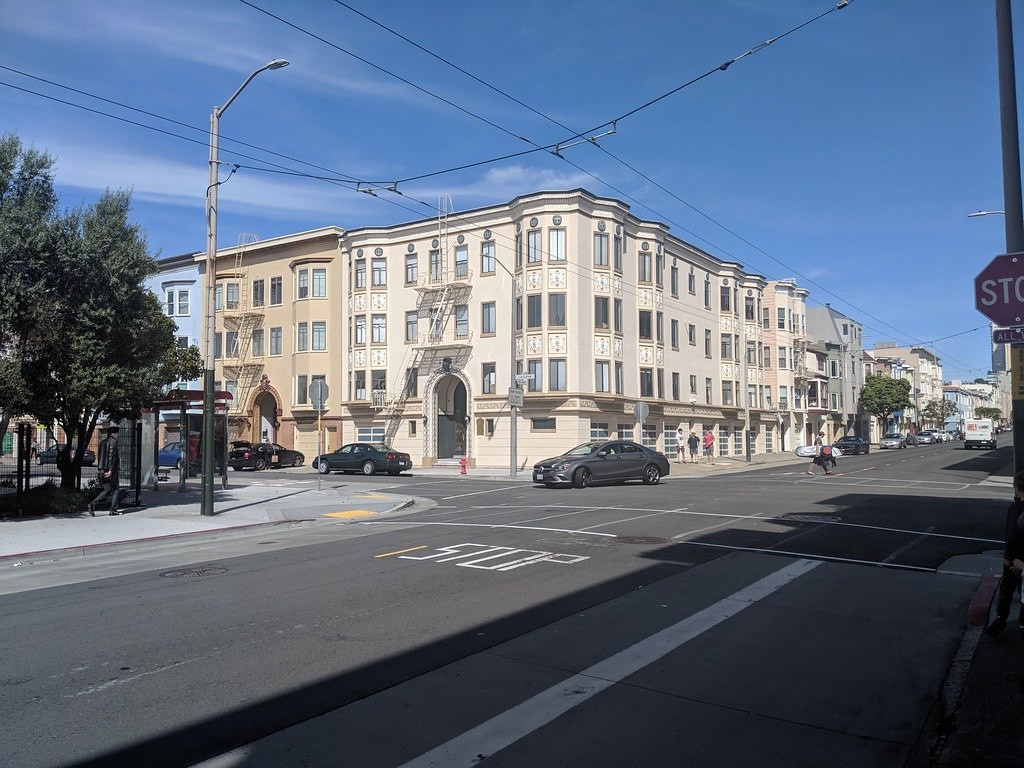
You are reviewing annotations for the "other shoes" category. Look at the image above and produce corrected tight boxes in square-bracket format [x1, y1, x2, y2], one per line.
[87, 504, 95, 517]
[984, 617, 1008, 637]
[807, 471, 816, 476]
[827, 471, 833, 475]
[110, 510, 124, 515]
[265, 467, 273, 471]
[683, 460, 686, 464]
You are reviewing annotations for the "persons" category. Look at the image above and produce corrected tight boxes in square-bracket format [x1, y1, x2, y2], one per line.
[808, 432, 832, 475]
[985, 472, 1024, 635]
[87, 426, 122, 516]
[676, 429, 687, 463]
[688, 432, 700, 463]
[30, 437, 37, 459]
[704, 429, 715, 465]
[610, 447, 616, 455]
[262, 440, 272, 470]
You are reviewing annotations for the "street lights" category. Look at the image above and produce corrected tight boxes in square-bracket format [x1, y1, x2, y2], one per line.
[201, 58, 291, 517]
[479, 252, 517, 477]
[841, 333, 872, 439]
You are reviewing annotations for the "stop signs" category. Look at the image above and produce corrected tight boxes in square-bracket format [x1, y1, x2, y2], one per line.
[973, 253, 1023, 327]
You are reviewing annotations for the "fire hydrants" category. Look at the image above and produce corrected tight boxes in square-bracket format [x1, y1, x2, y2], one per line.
[458, 457, 468, 474]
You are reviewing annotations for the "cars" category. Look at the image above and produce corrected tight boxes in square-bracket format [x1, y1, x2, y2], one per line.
[532, 440, 671, 489]
[312, 442, 413, 476]
[914, 427, 962, 445]
[831, 435, 870, 455]
[225, 440, 305, 471]
[879, 432, 907, 449]
[158, 441, 182, 470]
[34, 443, 95, 467]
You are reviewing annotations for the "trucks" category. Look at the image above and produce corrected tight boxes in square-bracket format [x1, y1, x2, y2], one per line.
[963, 416, 999, 449]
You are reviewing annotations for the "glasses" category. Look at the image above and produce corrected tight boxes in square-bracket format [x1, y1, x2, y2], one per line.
[1012, 483, 1023, 492]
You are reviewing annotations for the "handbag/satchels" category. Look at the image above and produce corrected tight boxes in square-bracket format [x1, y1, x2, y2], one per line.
[271, 455, 278, 463]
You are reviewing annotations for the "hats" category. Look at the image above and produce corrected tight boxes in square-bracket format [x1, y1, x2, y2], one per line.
[819, 431, 825, 435]
[107, 426, 120, 434]
